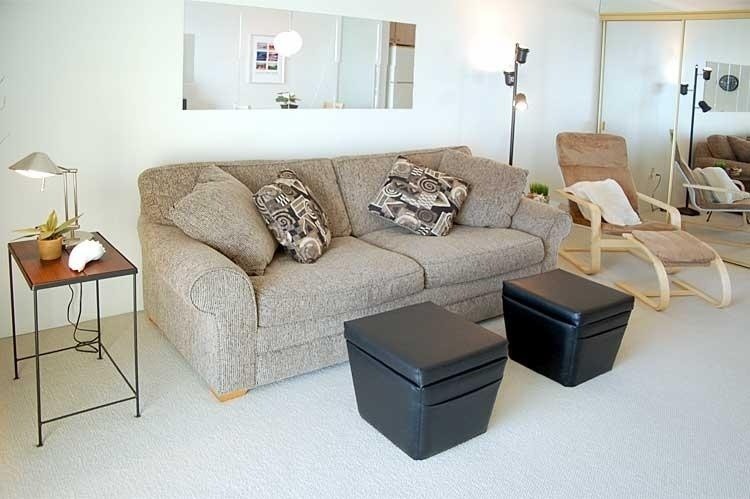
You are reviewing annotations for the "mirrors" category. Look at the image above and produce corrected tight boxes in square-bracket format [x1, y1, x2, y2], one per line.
[181, 0, 416, 111]
[595, 10, 750, 269]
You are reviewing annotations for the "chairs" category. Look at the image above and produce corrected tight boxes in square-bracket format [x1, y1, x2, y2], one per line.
[668, 130, 750, 225]
[553, 131, 732, 313]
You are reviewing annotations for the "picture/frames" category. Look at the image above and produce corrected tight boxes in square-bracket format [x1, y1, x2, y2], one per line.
[250, 33, 286, 84]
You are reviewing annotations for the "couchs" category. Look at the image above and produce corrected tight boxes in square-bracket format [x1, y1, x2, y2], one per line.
[692, 135, 750, 192]
[135, 145, 574, 404]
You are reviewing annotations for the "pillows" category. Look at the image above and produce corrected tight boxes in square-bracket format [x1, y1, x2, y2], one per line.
[366, 154, 471, 238]
[438, 147, 528, 231]
[706, 135, 736, 162]
[560, 177, 642, 227]
[171, 163, 280, 275]
[701, 165, 749, 202]
[726, 135, 750, 163]
[252, 168, 331, 262]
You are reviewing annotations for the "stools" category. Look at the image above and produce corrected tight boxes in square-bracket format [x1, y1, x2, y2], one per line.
[343, 299, 509, 461]
[501, 265, 636, 388]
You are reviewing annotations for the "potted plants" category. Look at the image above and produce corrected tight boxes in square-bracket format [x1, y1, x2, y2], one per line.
[274, 90, 299, 109]
[11, 211, 86, 262]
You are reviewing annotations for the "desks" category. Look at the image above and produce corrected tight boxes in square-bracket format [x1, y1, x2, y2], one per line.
[6, 229, 143, 449]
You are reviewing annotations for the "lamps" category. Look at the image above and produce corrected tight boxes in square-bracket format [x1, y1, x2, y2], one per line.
[503, 40, 531, 173]
[8, 151, 96, 250]
[677, 64, 713, 218]
[272, 3, 303, 57]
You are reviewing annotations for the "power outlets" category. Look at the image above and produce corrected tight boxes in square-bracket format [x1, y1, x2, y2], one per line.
[649, 167, 655, 181]
